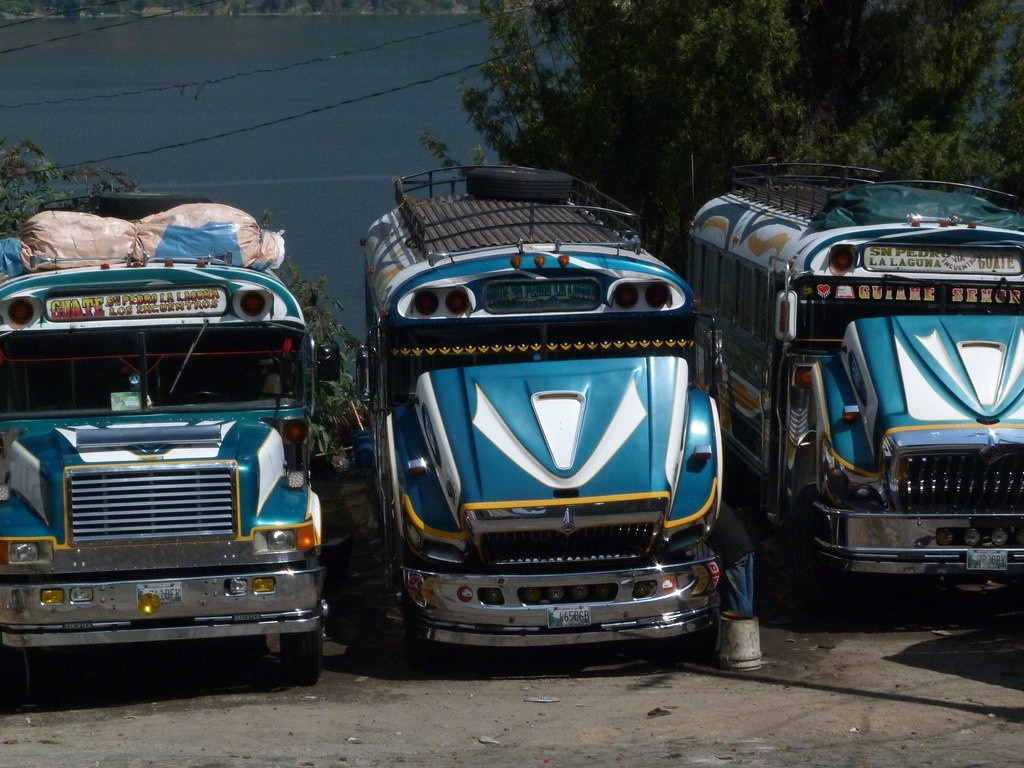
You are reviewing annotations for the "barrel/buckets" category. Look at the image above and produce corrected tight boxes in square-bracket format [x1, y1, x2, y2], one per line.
[719, 615, 762, 671]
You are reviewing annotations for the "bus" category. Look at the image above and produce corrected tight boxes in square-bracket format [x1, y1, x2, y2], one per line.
[354, 165, 723, 676]
[0, 193, 345, 688]
[686, 161, 1024, 617]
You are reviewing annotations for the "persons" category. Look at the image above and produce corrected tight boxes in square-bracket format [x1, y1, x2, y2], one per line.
[703, 498, 755, 615]
